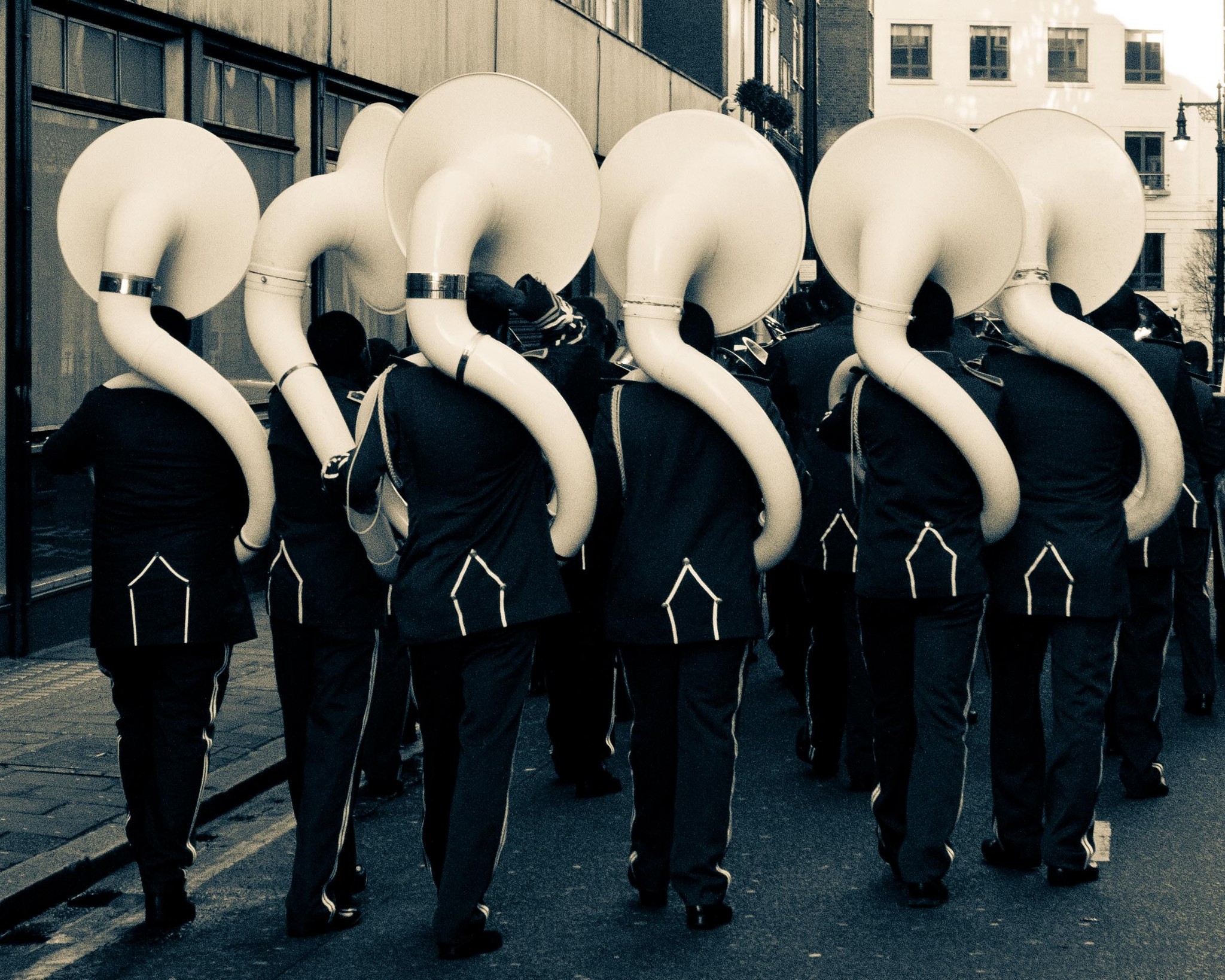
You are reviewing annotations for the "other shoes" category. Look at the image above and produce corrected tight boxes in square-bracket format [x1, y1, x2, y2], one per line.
[1047, 862, 1100, 887]
[328, 906, 361, 932]
[328, 864, 367, 897]
[436, 929, 503, 960]
[146, 901, 198, 933]
[686, 899, 733, 931]
[981, 838, 1042, 870]
[909, 876, 948, 908]
[575, 774, 621, 800]
[1184, 692, 1214, 717]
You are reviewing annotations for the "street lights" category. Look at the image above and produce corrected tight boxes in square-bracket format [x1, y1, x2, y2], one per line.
[1169, 86, 1225, 391]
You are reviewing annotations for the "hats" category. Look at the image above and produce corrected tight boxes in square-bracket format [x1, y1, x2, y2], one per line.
[1048, 281, 1084, 320]
[902, 278, 956, 346]
[678, 301, 715, 357]
[149, 304, 191, 348]
[464, 277, 510, 346]
[1183, 339, 1210, 370]
[567, 295, 618, 355]
[305, 311, 398, 392]
[1091, 284, 1142, 331]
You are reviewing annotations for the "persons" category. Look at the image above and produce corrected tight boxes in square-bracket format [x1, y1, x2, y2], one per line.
[348, 272, 602, 962]
[247, 310, 421, 938]
[21, 307, 260, 941]
[531, 279, 1225, 928]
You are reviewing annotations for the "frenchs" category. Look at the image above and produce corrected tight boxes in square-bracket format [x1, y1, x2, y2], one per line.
[54, 116, 277, 564]
[805, 115, 1028, 545]
[592, 106, 808, 573]
[384, 69, 604, 568]
[242, 99, 410, 476]
[971, 106, 1186, 541]
